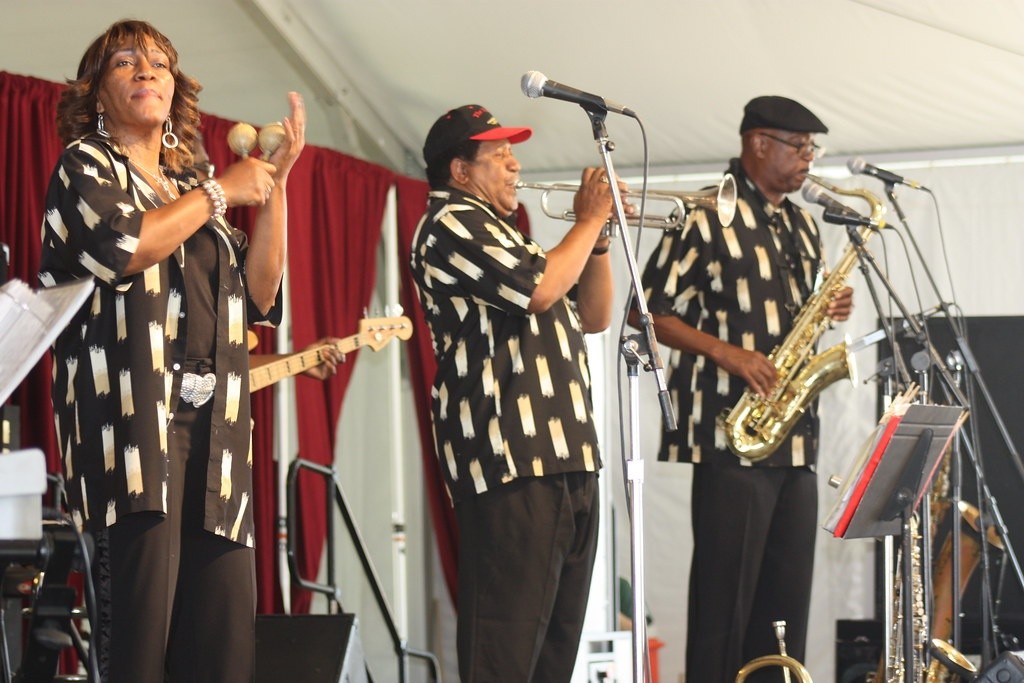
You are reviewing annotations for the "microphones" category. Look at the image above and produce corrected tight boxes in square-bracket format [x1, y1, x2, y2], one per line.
[803, 186, 877, 233]
[847, 157, 923, 191]
[520, 69, 636, 121]
[823, 207, 893, 230]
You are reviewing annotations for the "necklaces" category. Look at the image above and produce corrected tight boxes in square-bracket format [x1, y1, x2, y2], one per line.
[115, 159, 176, 200]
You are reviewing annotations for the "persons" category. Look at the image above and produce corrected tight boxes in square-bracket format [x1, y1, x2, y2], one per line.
[626, 96, 854, 683]
[36, 19, 307, 682]
[247, 336, 345, 381]
[408, 104, 635, 683]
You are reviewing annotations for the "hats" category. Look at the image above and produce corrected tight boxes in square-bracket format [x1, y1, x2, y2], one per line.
[423, 105, 532, 168]
[740, 96, 829, 134]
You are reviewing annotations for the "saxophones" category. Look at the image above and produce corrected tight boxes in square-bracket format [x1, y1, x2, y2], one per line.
[885, 510, 978, 683]
[722, 172, 885, 459]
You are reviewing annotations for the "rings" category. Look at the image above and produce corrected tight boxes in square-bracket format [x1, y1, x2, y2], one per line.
[600, 175, 610, 184]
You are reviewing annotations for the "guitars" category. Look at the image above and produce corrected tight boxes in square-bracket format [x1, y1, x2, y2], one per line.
[249, 302, 414, 394]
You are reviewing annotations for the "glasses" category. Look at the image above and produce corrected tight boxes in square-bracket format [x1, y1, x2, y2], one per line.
[760, 132, 822, 158]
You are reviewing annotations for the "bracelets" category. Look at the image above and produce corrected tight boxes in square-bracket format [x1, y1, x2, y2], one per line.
[201, 179, 227, 219]
[591, 239, 611, 255]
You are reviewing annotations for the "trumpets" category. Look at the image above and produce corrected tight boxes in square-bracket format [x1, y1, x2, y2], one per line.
[516, 172, 737, 239]
[734, 618, 813, 683]
